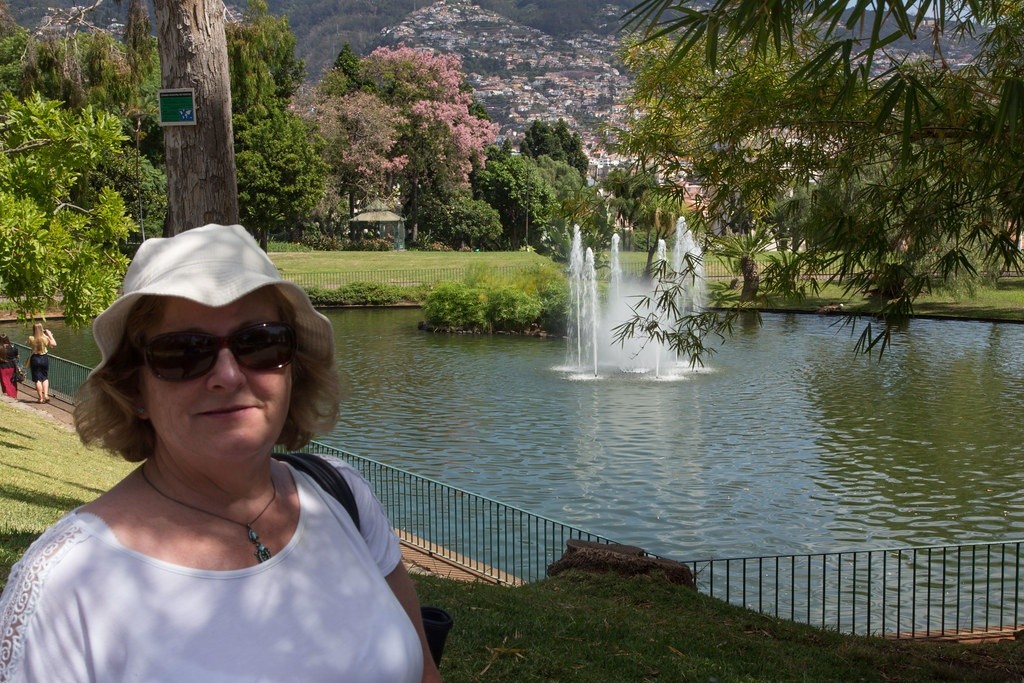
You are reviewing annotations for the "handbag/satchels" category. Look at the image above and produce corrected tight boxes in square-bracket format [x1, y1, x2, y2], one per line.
[416, 608, 454, 671]
[11, 362, 25, 383]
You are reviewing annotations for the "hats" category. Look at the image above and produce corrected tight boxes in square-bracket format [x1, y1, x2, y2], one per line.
[87, 223, 337, 385]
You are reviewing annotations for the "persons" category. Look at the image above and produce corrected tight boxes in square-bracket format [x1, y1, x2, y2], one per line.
[0, 223, 442, 683]
[27, 323, 57, 403]
[0, 334, 18, 399]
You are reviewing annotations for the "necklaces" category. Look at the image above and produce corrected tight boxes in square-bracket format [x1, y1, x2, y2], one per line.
[139, 462, 276, 563]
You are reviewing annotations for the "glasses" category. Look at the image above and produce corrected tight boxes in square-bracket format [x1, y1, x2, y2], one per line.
[139, 321, 299, 382]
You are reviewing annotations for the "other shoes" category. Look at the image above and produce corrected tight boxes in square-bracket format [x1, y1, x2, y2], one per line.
[36, 399, 44, 404]
[45, 399, 50, 404]
